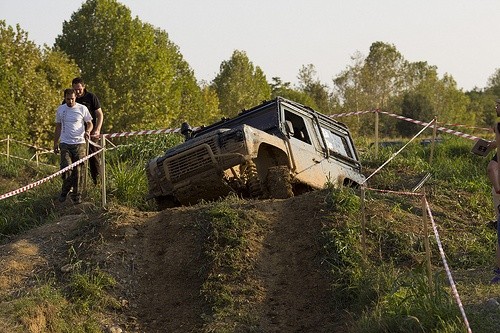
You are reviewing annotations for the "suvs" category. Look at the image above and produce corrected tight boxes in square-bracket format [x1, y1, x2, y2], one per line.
[143, 95, 367, 212]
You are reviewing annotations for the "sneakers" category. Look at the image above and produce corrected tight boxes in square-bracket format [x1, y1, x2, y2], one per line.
[74, 194, 81, 204]
[60, 191, 68, 200]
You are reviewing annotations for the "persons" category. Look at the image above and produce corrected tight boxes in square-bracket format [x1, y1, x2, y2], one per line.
[52, 88, 94, 206]
[61, 77, 104, 188]
